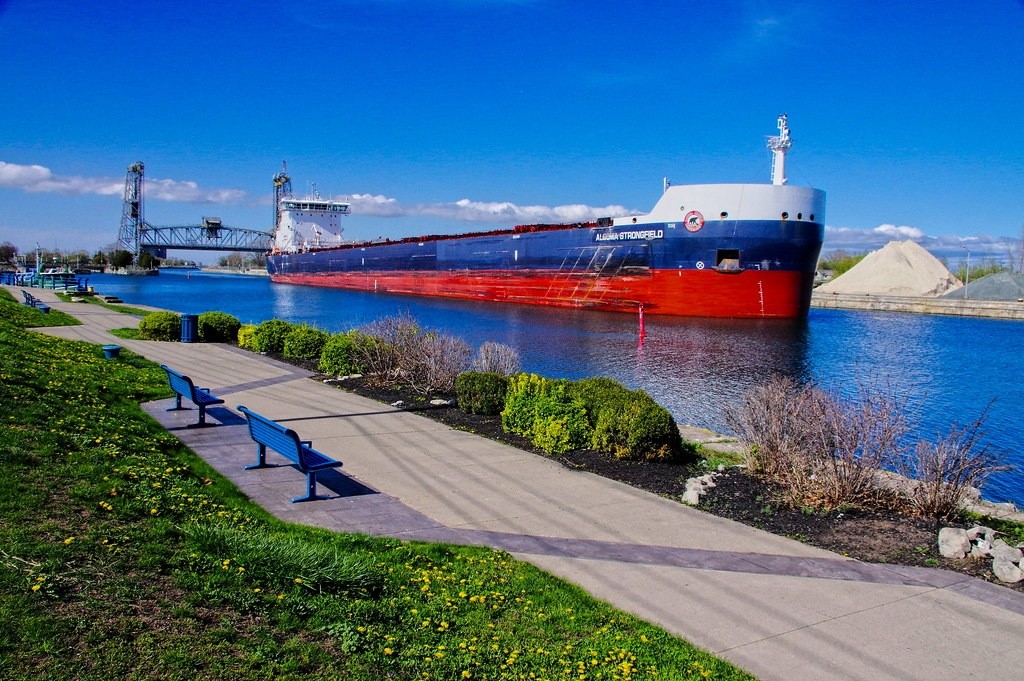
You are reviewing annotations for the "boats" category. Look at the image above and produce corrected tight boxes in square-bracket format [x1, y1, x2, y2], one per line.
[0, 242, 84, 290]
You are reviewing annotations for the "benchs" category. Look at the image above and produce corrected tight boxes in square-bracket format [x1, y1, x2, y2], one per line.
[236, 405, 344, 503]
[160, 364, 226, 430]
[20, 289, 52, 314]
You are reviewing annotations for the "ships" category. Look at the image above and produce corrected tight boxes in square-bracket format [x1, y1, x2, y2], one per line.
[265, 111, 827, 319]
